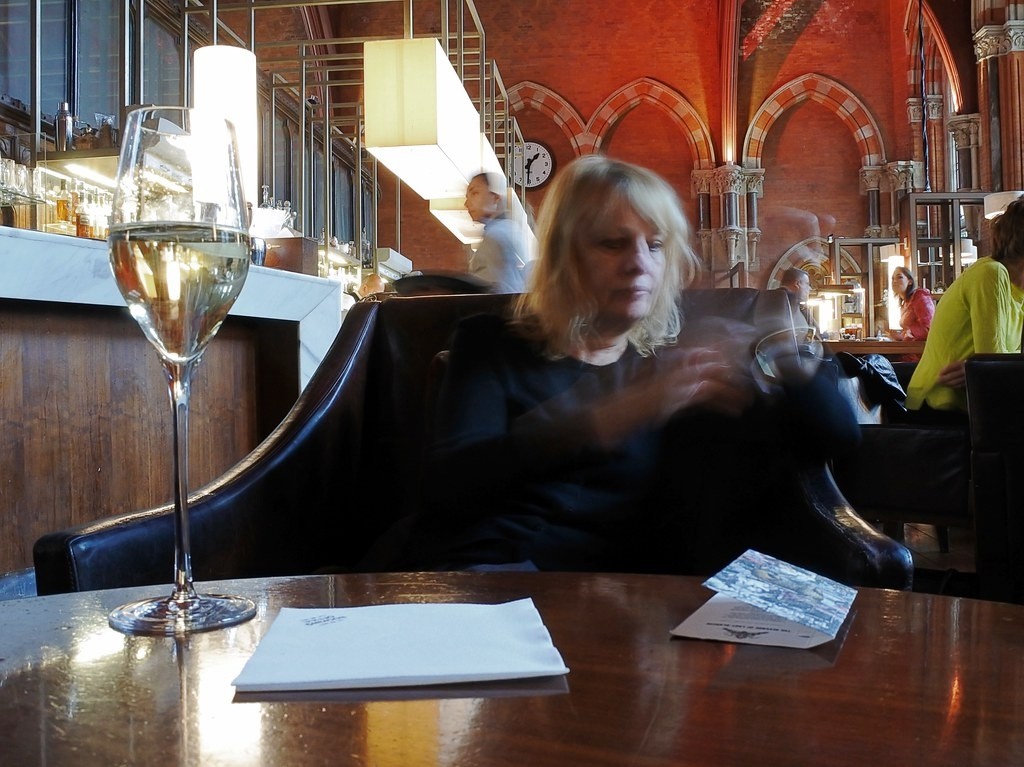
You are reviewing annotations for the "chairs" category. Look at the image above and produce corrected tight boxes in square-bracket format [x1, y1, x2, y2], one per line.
[31, 287, 1024, 599]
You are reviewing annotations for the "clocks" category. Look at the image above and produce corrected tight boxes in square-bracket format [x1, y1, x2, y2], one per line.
[503, 138, 555, 191]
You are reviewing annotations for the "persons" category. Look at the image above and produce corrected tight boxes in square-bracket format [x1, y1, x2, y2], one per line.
[882, 267, 934, 343]
[418, 157, 860, 573]
[906, 195, 1023, 414]
[464, 171, 526, 294]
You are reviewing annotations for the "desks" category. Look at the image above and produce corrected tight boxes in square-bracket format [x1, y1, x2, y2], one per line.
[822, 340, 927, 356]
[0, 569, 1024, 767]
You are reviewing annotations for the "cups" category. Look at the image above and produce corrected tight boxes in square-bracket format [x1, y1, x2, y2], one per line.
[0, 158, 43, 207]
[840, 324, 862, 340]
[250, 237, 266, 266]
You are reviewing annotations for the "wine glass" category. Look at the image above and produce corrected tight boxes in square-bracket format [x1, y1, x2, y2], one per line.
[107, 107, 256, 635]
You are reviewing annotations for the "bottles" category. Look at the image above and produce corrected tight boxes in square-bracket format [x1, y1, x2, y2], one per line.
[54, 102, 73, 151]
[319, 227, 371, 292]
[57, 178, 112, 240]
[259, 185, 297, 230]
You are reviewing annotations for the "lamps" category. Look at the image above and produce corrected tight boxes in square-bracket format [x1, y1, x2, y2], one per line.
[362, 1, 539, 267]
